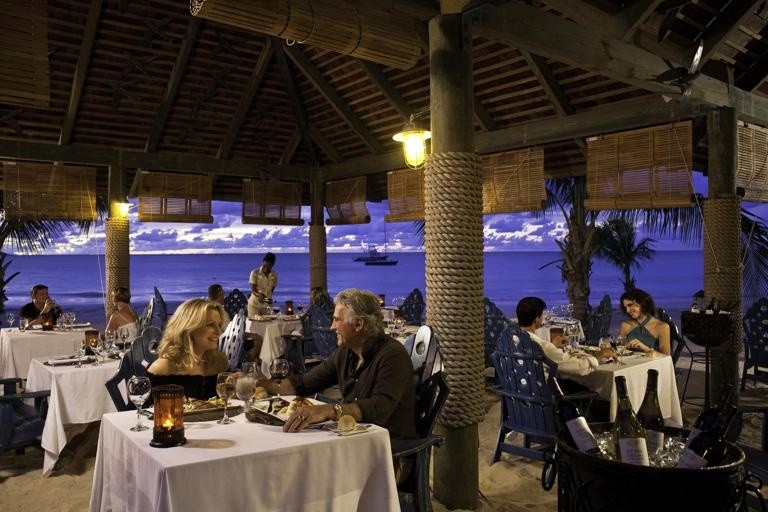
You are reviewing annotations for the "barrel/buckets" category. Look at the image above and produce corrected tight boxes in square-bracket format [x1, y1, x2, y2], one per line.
[554, 421, 746, 511]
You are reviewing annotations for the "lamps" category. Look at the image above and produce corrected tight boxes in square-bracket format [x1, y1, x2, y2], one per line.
[113, 193, 134, 218]
[657, 17, 704, 84]
[391, 111, 432, 172]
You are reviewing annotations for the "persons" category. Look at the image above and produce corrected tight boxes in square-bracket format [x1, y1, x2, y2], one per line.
[513, 297, 616, 395]
[141, 298, 230, 404]
[620, 289, 671, 355]
[20, 285, 63, 329]
[248, 251, 277, 314]
[104, 287, 140, 348]
[226, 288, 418, 488]
[302, 287, 335, 334]
[209, 284, 231, 329]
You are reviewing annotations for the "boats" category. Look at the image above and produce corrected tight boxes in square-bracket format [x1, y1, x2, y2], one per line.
[353, 246, 398, 265]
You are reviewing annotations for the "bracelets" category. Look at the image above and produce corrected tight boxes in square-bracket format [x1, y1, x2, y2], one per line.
[647, 347, 654, 354]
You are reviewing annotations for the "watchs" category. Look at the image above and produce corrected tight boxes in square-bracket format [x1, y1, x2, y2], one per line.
[332, 403, 343, 424]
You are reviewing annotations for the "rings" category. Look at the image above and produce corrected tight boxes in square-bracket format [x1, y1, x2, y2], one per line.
[298, 415, 304, 420]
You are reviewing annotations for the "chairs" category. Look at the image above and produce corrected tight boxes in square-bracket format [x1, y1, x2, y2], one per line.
[222, 289, 248, 320]
[154, 286, 167, 326]
[139, 297, 155, 329]
[219, 308, 246, 372]
[658, 307, 686, 366]
[740, 297, 768, 392]
[106, 328, 166, 412]
[487, 324, 598, 482]
[399, 288, 424, 326]
[310, 305, 337, 356]
[393, 326, 450, 512]
[484, 297, 512, 383]
[578, 295, 612, 345]
[0, 377, 51, 452]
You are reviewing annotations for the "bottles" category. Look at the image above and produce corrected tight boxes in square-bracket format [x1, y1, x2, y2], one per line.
[690, 300, 700, 314]
[705, 297, 716, 315]
[546, 368, 739, 469]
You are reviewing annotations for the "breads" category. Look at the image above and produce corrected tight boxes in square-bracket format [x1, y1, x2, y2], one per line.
[255, 387, 268, 399]
[338, 414, 357, 430]
[278, 397, 314, 416]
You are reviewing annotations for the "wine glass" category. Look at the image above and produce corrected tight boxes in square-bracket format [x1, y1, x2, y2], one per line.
[296, 302, 303, 313]
[6, 311, 76, 333]
[126, 358, 290, 432]
[272, 307, 280, 315]
[549, 303, 629, 367]
[388, 324, 395, 338]
[73, 327, 129, 368]
